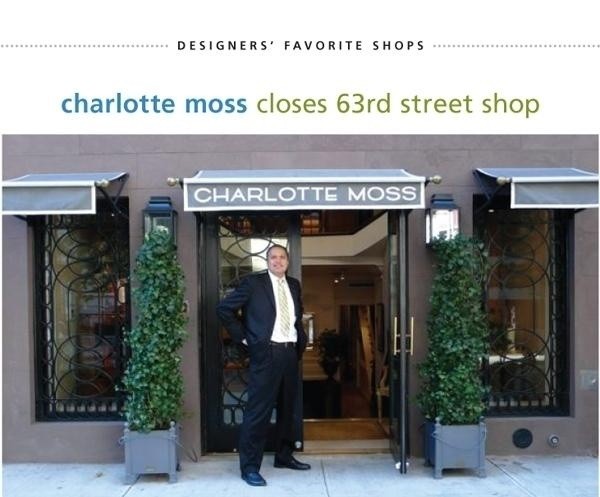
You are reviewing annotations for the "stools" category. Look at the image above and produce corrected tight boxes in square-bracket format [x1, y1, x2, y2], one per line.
[270, 342, 300, 349]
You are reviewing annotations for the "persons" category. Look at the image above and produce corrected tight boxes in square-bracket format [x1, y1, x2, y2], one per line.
[216, 244, 311, 487]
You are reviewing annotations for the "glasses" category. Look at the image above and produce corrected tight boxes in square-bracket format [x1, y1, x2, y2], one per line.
[425, 193, 460, 247]
[141, 196, 178, 248]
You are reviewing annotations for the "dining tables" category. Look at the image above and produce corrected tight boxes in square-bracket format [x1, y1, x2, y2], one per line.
[115, 229, 189, 485]
[417, 229, 491, 479]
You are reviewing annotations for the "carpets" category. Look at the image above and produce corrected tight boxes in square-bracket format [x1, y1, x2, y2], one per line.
[276, 279, 290, 338]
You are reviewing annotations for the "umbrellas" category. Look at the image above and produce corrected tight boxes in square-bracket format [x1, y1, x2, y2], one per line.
[273, 454, 311, 470]
[241, 472, 266, 486]
